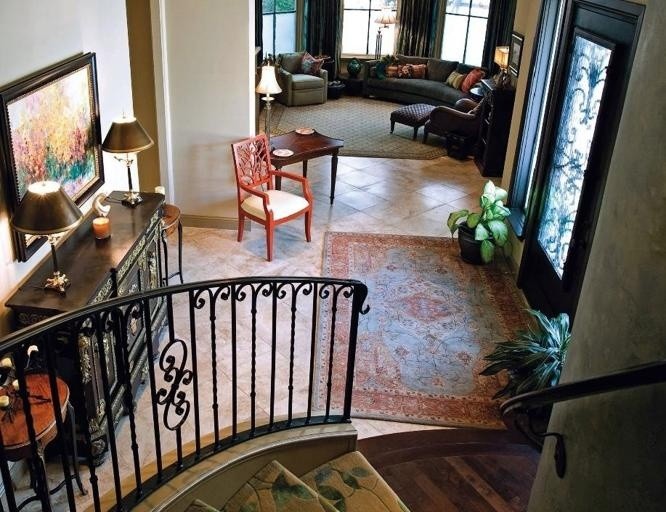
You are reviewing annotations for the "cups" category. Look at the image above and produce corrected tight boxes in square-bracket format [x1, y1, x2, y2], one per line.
[91, 217, 111, 241]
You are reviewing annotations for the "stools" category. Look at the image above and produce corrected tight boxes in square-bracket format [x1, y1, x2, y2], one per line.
[328, 83, 345, 98]
[389, 103, 435, 140]
[162, 202, 184, 288]
[0, 374, 91, 512]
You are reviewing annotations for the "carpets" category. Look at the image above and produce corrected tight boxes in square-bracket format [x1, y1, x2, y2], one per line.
[258, 96, 448, 160]
[309, 229, 540, 430]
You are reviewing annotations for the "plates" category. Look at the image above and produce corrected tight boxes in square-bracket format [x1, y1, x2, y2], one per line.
[272, 148, 294, 159]
[295, 127, 314, 136]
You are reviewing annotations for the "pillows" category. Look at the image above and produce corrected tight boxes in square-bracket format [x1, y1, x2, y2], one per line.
[459, 66, 485, 94]
[384, 62, 426, 79]
[296, 51, 324, 76]
[443, 69, 467, 89]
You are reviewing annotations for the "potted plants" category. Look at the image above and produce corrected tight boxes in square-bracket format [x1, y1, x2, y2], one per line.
[446, 179, 513, 267]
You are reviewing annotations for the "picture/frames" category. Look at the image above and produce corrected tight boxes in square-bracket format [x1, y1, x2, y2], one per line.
[0, 50, 105, 263]
[507, 31, 524, 77]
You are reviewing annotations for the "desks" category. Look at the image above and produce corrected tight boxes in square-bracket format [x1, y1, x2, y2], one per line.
[254, 128, 344, 205]
[338, 72, 364, 97]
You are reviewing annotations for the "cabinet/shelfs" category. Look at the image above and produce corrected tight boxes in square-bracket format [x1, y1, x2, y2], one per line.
[472, 79, 515, 178]
[5, 189, 167, 466]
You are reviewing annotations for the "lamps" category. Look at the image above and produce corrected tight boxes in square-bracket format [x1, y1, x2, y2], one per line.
[255, 59, 282, 154]
[493, 45, 509, 88]
[100, 107, 153, 207]
[8, 179, 85, 296]
[373, 7, 399, 59]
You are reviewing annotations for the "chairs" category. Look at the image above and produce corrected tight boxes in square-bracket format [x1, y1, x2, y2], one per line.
[422, 94, 490, 160]
[271, 50, 328, 107]
[230, 133, 313, 262]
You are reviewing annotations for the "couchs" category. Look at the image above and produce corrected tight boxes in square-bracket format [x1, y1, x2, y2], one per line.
[363, 54, 490, 109]
